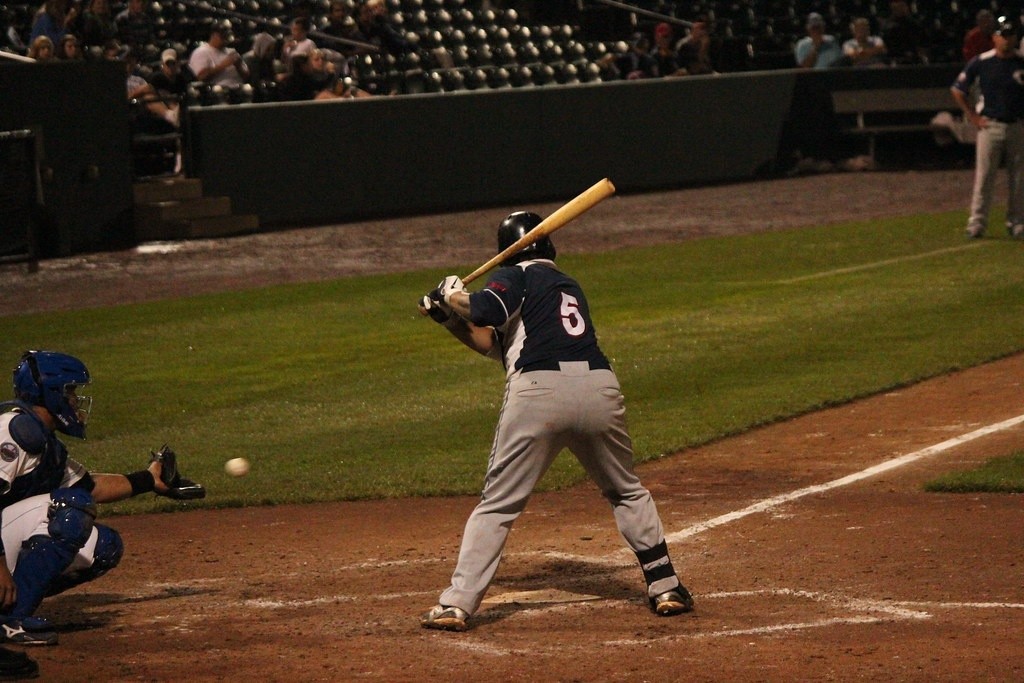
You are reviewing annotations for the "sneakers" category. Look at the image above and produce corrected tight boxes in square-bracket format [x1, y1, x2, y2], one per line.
[420, 604, 470, 632]
[1004, 222, 1024, 237]
[653, 590, 686, 616]
[0, 620, 59, 647]
[967, 223, 987, 237]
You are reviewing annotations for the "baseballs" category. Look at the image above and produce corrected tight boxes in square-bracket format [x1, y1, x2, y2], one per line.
[225, 457, 250, 477]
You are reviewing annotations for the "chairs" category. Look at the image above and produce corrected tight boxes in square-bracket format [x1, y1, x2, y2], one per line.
[0, 0, 1024, 172]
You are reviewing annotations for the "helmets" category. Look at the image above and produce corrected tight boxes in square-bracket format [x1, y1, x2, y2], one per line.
[497, 211, 557, 263]
[990, 16, 1021, 37]
[12, 349, 93, 442]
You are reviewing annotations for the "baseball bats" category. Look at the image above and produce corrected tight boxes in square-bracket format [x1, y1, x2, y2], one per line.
[417, 177, 616, 317]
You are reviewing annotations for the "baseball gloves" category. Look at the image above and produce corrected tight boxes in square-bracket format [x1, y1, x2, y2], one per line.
[149, 442, 206, 500]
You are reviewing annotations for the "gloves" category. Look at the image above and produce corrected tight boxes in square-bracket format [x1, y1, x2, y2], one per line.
[437, 274, 467, 298]
[420, 290, 452, 324]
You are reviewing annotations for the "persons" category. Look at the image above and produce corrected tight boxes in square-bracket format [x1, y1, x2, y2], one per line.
[962, 10, 997, 61]
[0, 350, 207, 644]
[796, 11, 845, 67]
[0, 0, 420, 128]
[949, 13, 1024, 238]
[417, 211, 693, 627]
[840, 15, 890, 66]
[598, 16, 719, 81]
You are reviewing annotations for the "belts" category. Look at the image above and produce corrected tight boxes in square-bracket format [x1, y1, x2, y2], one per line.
[519, 362, 612, 373]
